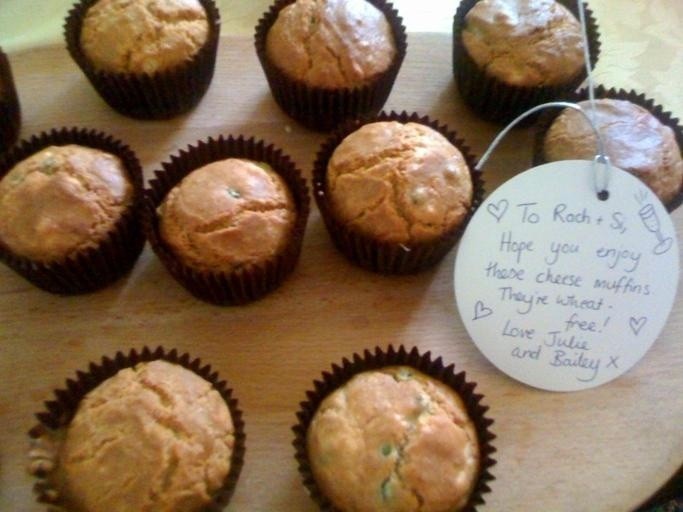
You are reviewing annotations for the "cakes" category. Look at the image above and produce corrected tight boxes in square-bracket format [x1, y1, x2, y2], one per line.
[156, 158, 299, 273]
[544, 98, 683, 207]
[308, 365, 482, 512]
[59, 360, 235, 511]
[327, 121, 473, 245]
[266, 1, 397, 90]
[461, 0, 589, 86]
[80, 0, 209, 73]
[0, 144, 133, 262]
